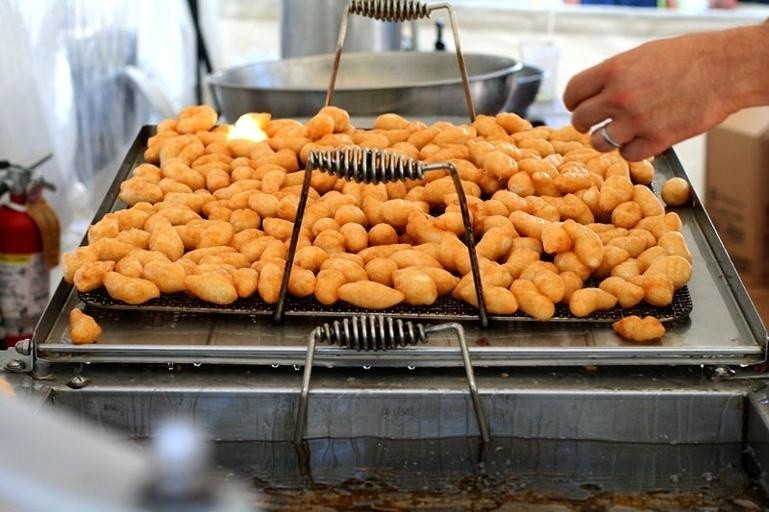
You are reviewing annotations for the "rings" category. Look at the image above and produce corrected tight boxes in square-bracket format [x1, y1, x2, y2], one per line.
[600, 126, 623, 148]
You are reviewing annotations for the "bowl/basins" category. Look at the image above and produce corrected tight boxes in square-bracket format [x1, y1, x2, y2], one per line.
[205, 50, 524, 125]
[508, 64, 541, 117]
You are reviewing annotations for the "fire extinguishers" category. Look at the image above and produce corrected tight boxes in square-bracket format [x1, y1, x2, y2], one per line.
[0, 153, 60, 351]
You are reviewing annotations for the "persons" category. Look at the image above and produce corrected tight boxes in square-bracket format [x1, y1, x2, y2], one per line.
[563, 22, 768, 164]
